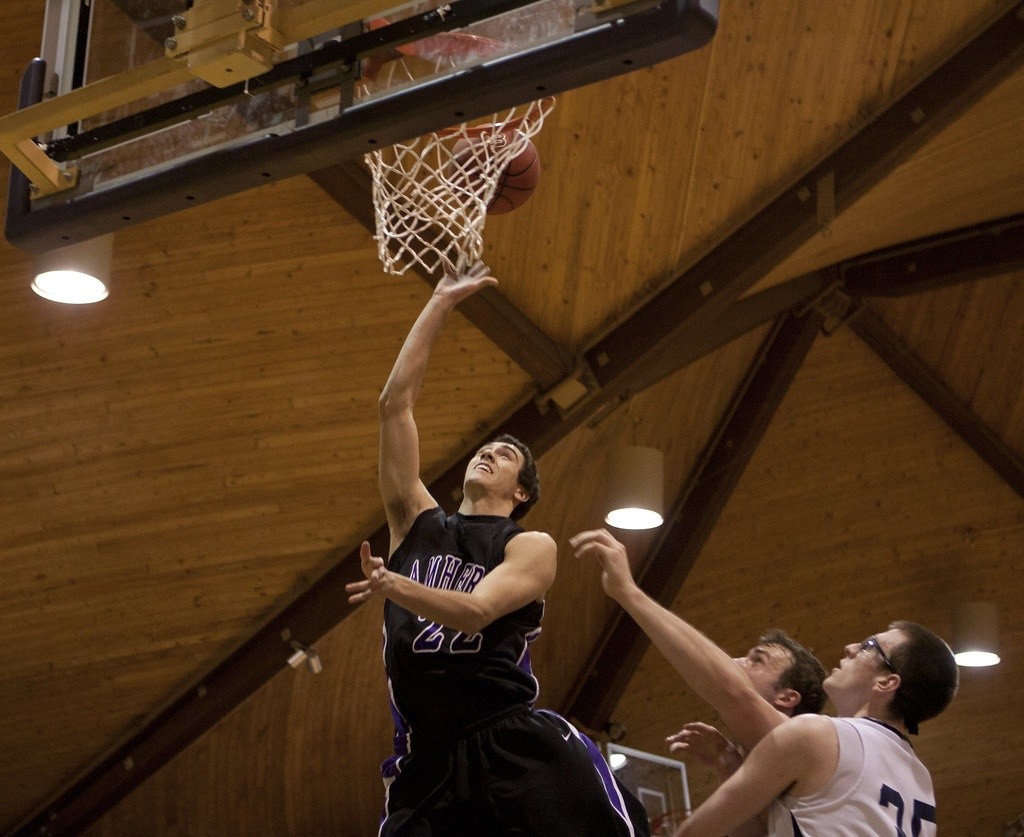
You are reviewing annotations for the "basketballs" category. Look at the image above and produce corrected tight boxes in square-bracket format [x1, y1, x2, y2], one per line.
[450, 124, 542, 217]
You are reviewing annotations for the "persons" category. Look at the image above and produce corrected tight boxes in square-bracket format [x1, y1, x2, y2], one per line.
[345, 260, 652, 837]
[568, 528, 958, 837]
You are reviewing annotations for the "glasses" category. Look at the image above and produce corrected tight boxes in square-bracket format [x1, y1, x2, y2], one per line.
[860, 634, 893, 674]
